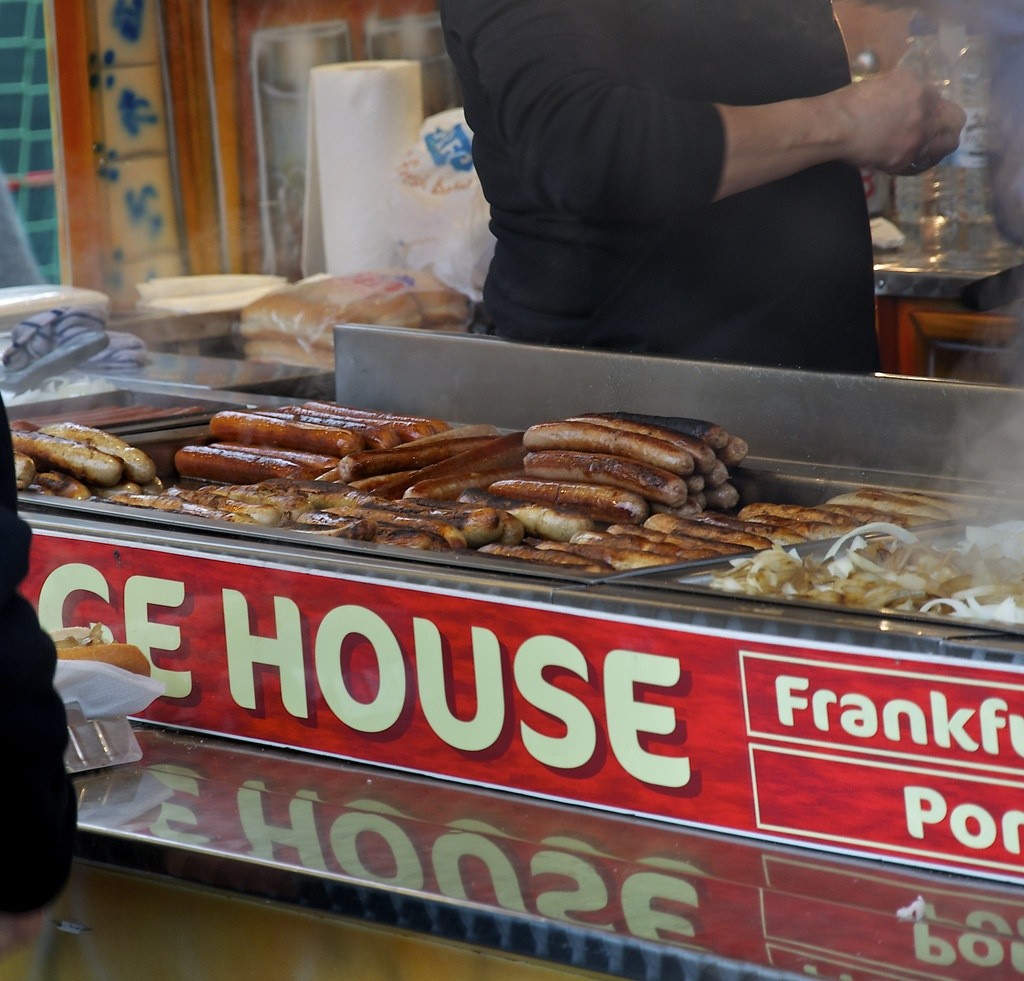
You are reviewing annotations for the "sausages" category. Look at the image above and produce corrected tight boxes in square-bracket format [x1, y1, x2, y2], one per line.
[8, 399, 990, 576]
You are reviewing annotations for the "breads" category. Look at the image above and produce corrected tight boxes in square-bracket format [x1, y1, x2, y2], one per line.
[237, 267, 472, 370]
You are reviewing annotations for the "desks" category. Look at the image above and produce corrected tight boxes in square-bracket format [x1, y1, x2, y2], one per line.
[874, 222, 1023, 371]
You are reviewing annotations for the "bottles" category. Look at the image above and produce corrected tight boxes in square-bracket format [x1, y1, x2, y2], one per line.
[950, 20, 995, 225]
[891, 14, 959, 252]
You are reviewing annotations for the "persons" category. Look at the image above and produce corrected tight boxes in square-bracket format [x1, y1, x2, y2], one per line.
[440, 0, 1024, 378]
[0, 392, 78, 961]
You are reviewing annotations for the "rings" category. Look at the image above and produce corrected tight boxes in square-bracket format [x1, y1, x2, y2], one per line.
[912, 158, 921, 170]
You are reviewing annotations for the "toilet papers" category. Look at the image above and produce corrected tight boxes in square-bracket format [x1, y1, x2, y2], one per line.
[298, 59, 424, 279]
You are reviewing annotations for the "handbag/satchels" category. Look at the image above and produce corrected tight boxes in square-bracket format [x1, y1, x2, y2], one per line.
[380, 107, 497, 303]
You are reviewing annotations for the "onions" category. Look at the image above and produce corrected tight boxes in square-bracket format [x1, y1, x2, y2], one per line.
[704, 519, 1024, 630]
